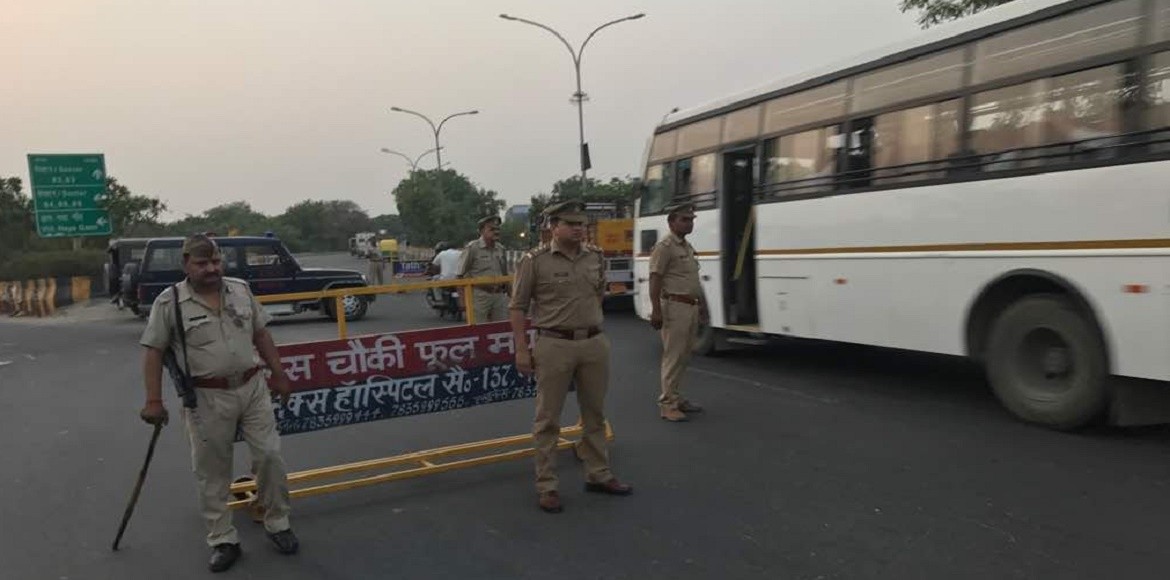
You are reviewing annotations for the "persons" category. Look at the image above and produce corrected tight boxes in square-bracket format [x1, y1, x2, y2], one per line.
[365, 240, 385, 285]
[138, 236, 299, 572]
[455, 216, 514, 324]
[649, 202, 702, 421]
[509, 199, 633, 512]
[419, 241, 463, 308]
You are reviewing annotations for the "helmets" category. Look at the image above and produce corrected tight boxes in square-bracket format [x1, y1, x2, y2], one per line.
[435, 241, 448, 251]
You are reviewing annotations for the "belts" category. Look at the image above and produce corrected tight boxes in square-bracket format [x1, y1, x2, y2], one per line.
[538, 326, 603, 340]
[473, 283, 506, 293]
[190, 366, 260, 389]
[663, 294, 703, 306]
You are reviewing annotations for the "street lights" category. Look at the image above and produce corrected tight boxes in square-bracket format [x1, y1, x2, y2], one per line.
[390, 106, 480, 178]
[498, 12, 649, 211]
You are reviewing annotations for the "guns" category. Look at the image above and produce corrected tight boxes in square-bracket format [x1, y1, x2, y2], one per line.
[161, 346, 198, 409]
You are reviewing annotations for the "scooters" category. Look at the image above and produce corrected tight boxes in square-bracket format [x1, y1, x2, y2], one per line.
[425, 270, 465, 321]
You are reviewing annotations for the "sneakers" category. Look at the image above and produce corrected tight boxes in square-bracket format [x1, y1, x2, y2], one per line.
[661, 409, 687, 421]
[679, 400, 703, 412]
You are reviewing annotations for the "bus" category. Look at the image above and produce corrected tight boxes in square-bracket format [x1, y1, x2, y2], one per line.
[633, 0, 1170, 424]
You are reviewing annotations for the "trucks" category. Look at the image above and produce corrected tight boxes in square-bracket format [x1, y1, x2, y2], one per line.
[527, 200, 635, 309]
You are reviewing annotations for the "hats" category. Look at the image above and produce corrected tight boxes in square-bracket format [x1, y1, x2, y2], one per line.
[544, 198, 589, 223]
[184, 232, 217, 257]
[664, 200, 697, 219]
[478, 214, 502, 226]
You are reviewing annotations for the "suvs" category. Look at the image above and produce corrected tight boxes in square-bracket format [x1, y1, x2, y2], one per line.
[108, 237, 150, 313]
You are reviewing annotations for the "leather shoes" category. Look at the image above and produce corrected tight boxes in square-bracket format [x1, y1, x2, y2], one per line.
[539, 490, 562, 514]
[265, 527, 298, 554]
[586, 477, 633, 495]
[209, 544, 242, 570]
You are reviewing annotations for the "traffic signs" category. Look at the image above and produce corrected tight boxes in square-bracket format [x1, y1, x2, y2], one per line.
[26, 152, 115, 239]
[117, 230, 377, 324]
